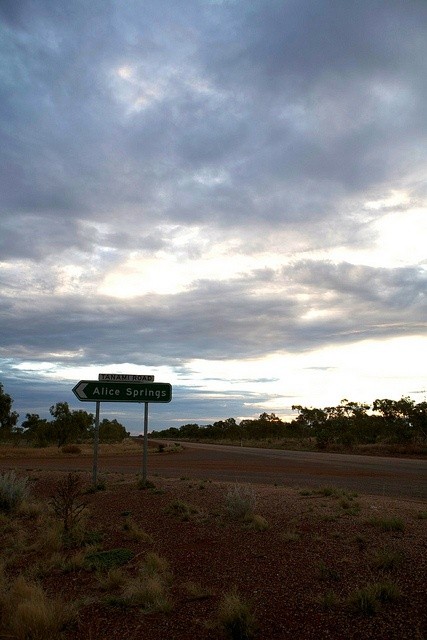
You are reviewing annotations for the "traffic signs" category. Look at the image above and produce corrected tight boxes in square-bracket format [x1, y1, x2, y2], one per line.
[72, 373, 172, 403]
